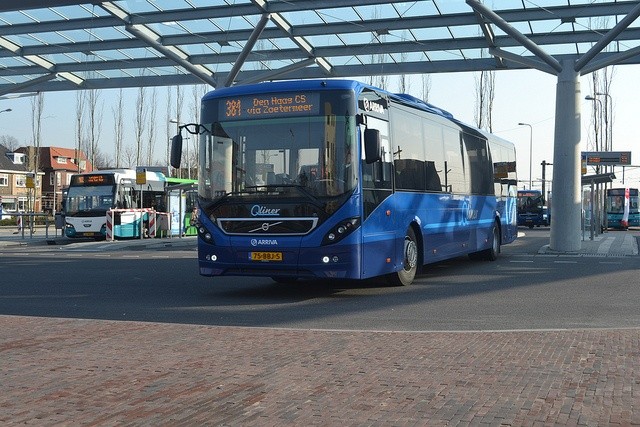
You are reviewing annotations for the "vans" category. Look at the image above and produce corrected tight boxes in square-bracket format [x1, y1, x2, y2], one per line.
[538, 206, 548, 227]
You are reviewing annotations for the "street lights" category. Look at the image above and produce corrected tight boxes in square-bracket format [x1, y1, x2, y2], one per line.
[594, 93, 612, 189]
[169, 119, 191, 180]
[586, 95, 602, 235]
[519, 123, 532, 191]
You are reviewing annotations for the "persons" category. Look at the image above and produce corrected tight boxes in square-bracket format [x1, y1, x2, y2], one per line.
[190, 206, 198, 228]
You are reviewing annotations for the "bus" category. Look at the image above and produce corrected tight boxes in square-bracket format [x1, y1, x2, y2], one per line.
[170, 80, 518, 287]
[518, 190, 542, 229]
[607, 188, 640, 229]
[166, 177, 198, 236]
[64, 169, 168, 241]
